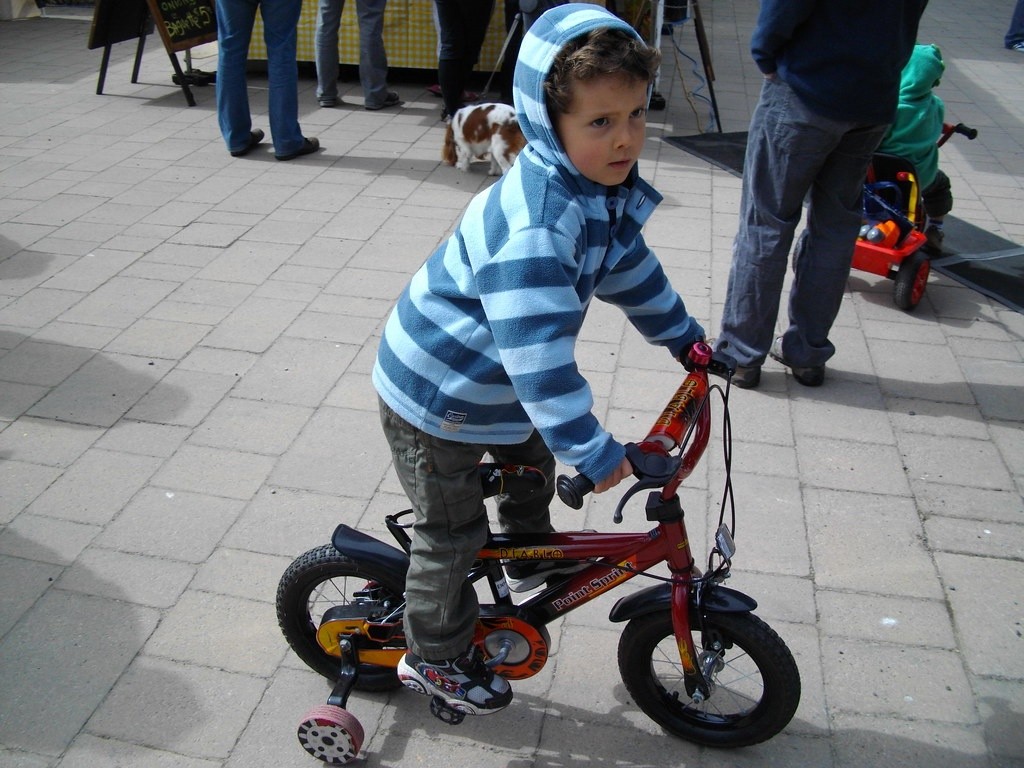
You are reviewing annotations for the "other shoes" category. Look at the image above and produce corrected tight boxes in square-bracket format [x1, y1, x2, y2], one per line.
[318, 96, 336, 106]
[923, 225, 943, 252]
[704, 338, 761, 389]
[364, 90, 400, 109]
[275, 137, 319, 161]
[231, 128, 265, 155]
[1007, 40, 1023, 52]
[768, 334, 825, 387]
[440, 104, 464, 122]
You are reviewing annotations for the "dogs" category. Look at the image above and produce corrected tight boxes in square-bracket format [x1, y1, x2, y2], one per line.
[442, 103, 528, 175]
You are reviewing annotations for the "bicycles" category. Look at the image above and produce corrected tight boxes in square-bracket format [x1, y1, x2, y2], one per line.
[276, 339, 805, 765]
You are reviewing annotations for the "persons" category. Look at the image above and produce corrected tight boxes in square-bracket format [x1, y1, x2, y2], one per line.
[705, 0, 928, 388]
[214, 0, 320, 160]
[874, 43, 953, 251]
[1005, 0, 1024, 51]
[374, 4, 706, 715]
[605, 0, 665, 109]
[429, 0, 524, 120]
[314, 0, 399, 109]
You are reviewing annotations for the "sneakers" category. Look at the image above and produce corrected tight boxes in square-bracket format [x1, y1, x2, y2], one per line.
[504, 530, 597, 593]
[396, 648, 513, 716]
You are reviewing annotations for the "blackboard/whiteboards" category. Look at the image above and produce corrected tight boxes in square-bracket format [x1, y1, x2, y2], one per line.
[87, 0, 218, 52]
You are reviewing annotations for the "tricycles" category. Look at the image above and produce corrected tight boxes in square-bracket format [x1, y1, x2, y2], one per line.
[790, 118, 982, 315]
